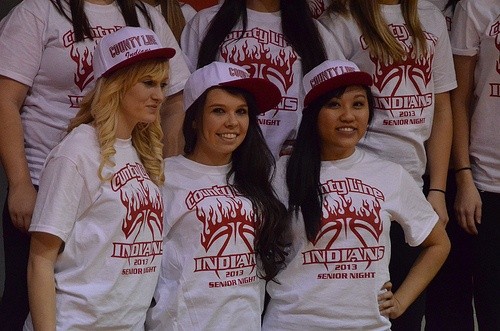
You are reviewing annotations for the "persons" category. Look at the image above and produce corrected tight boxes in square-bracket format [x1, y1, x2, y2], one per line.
[315, 0, 458, 331]
[139, 0, 459, 163]
[145, 62, 294, 331]
[424, 0, 500, 331]
[0, 0, 192, 331]
[21, 26, 165, 331]
[253, 58, 451, 331]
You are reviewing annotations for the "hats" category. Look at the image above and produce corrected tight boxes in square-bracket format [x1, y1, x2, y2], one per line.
[302, 59, 373, 111]
[182, 59, 282, 117]
[93, 26, 177, 81]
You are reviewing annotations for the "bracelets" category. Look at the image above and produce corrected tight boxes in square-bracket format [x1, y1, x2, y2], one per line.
[455, 167, 472, 174]
[428, 188, 446, 195]
